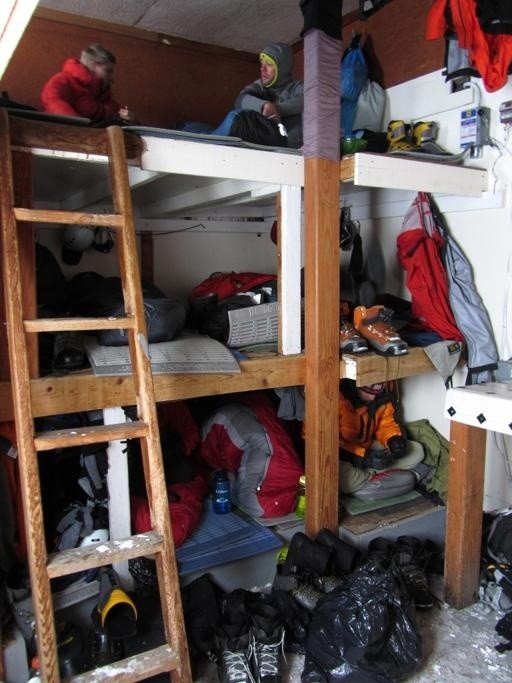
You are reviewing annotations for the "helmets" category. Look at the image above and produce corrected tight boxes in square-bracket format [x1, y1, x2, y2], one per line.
[340, 139, 368, 153]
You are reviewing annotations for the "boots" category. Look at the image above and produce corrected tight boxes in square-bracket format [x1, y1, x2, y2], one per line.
[387, 120, 425, 152]
[413, 121, 446, 153]
[339, 303, 368, 353]
[352, 305, 408, 356]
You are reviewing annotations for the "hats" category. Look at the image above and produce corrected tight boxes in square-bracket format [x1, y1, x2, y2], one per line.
[61, 226, 95, 265]
[359, 382, 385, 395]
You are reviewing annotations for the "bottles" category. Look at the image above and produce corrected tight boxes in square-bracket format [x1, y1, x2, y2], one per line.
[211, 470, 232, 514]
[296, 475, 306, 517]
[24, 656, 43, 682]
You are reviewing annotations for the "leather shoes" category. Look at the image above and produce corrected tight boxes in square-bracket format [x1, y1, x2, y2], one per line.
[53, 332, 87, 370]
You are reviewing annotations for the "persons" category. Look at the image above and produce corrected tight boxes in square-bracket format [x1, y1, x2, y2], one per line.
[39, 42, 137, 124]
[210, 42, 303, 149]
[339, 381, 430, 503]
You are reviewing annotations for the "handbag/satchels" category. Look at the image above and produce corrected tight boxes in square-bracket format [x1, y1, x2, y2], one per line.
[481, 506, 511, 567]
[341, 33, 386, 138]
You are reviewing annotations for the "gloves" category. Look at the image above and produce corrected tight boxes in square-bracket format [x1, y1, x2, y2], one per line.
[367, 451, 394, 470]
[387, 435, 407, 458]
